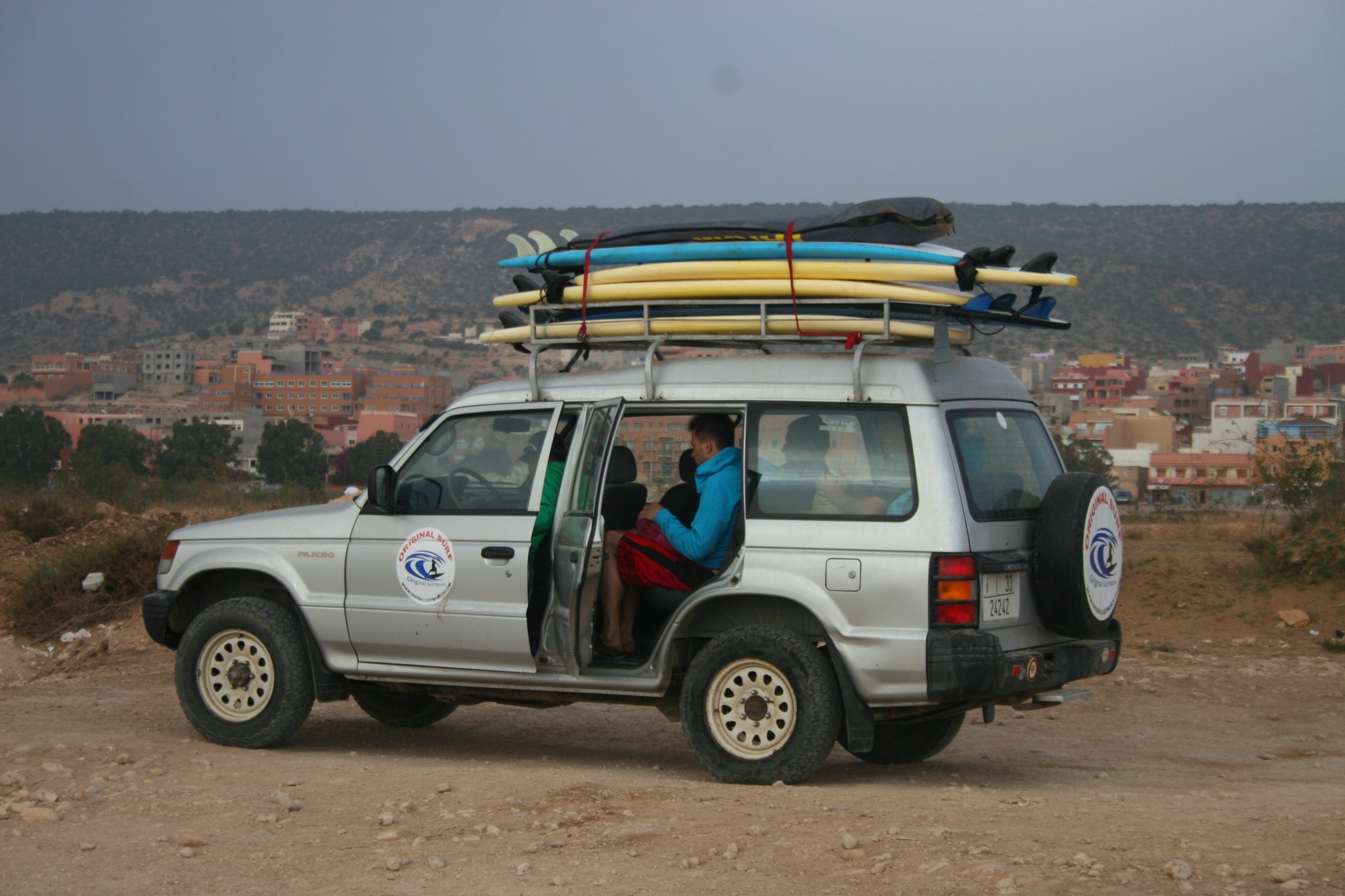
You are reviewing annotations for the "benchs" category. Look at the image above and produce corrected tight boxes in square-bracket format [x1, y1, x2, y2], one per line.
[888, 474, 1024, 516]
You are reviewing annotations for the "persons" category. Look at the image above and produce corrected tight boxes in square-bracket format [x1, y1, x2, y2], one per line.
[763, 414, 831, 516]
[516, 429, 589, 564]
[593, 412, 746, 659]
[816, 413, 910, 515]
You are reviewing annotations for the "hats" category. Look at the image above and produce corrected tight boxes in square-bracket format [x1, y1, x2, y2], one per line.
[516, 431, 562, 463]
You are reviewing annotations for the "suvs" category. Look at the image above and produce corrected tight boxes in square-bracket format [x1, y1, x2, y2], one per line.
[140, 341, 1125, 787]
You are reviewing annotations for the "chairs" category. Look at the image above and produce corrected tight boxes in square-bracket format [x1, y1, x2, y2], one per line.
[661, 449, 700, 530]
[601, 444, 647, 531]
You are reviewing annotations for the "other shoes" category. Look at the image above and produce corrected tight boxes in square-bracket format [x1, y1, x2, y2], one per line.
[625, 652, 641, 663]
[592, 643, 629, 661]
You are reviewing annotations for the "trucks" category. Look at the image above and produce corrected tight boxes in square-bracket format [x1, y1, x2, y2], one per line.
[1115, 490, 1134, 506]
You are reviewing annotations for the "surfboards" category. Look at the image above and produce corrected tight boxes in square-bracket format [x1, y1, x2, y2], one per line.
[480, 228, 1078, 341]
